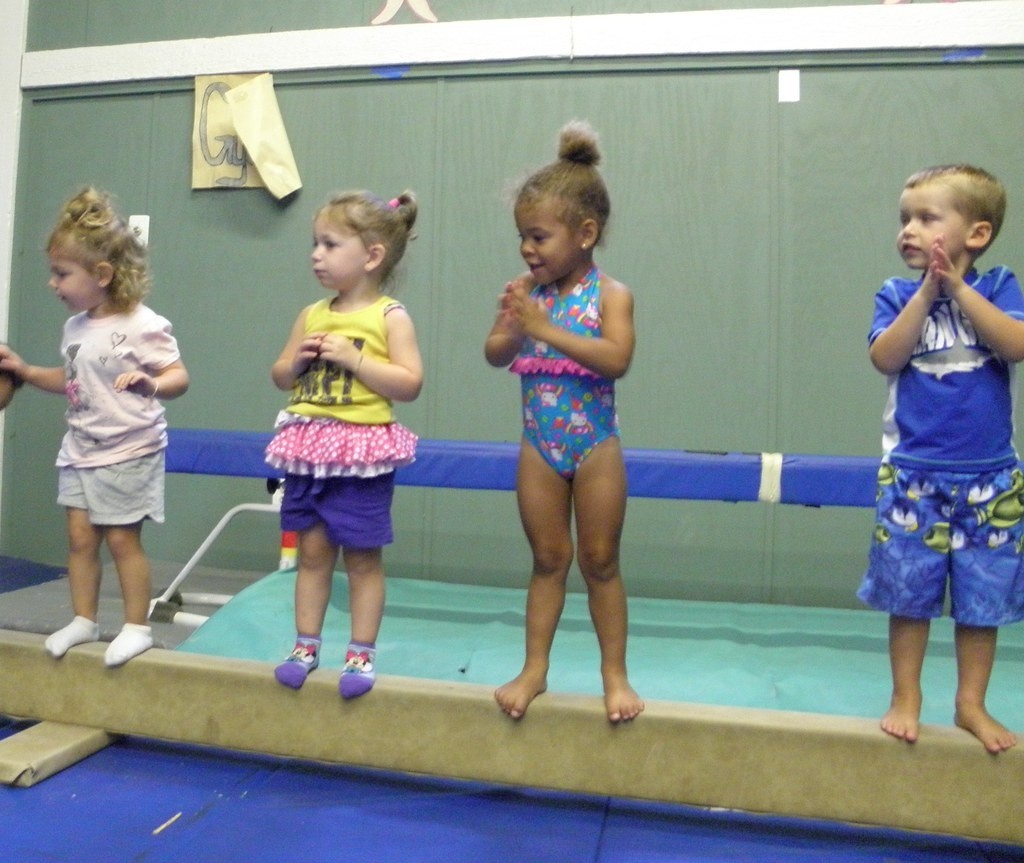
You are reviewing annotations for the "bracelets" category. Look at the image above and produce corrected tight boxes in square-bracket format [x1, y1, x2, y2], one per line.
[354, 352, 364, 376]
[142, 378, 159, 399]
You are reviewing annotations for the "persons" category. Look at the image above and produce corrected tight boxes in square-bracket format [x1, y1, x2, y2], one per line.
[484, 119, 644, 723]
[0, 186, 191, 666]
[858, 166, 1024, 753]
[264, 188, 423, 696]
